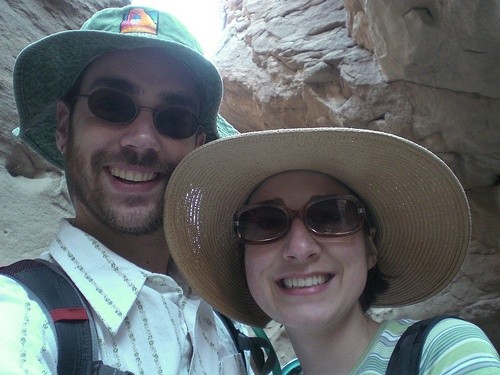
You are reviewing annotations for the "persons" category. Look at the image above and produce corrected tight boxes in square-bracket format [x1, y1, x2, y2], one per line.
[162, 127, 500, 374]
[1, 5, 271, 375]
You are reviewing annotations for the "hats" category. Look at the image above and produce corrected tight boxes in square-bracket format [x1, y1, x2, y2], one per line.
[163, 127, 473, 332]
[12, 7, 222, 172]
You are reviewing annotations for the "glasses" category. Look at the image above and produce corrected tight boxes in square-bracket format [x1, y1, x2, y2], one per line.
[234, 192, 374, 245]
[69, 91, 206, 140]
